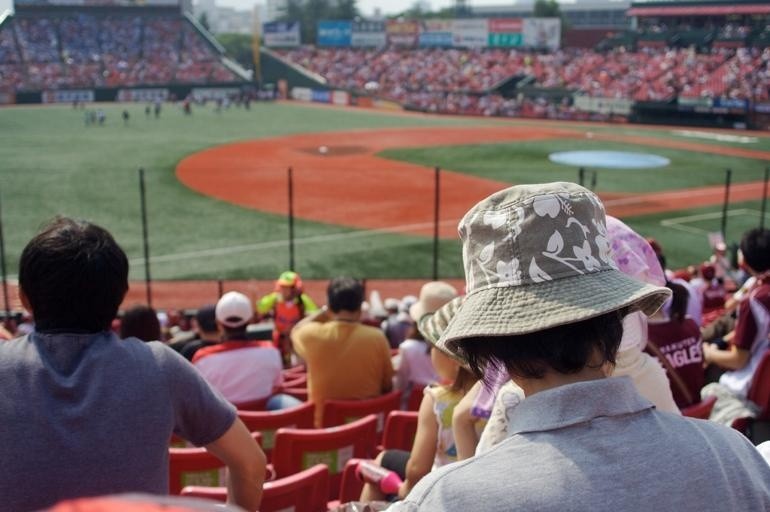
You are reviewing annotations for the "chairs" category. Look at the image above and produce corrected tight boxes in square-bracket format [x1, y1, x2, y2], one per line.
[0, 9, 250, 92]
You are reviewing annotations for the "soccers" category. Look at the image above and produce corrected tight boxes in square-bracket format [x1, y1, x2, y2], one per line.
[319, 146, 327, 153]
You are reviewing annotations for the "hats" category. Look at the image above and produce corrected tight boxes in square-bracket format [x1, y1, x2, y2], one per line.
[435, 181, 673, 367]
[417, 293, 485, 376]
[215, 291, 252, 328]
[408, 281, 458, 324]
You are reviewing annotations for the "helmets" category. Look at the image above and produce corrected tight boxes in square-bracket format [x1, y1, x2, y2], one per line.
[275, 272, 303, 298]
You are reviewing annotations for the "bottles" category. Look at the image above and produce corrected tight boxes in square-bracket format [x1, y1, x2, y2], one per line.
[356, 461, 403, 497]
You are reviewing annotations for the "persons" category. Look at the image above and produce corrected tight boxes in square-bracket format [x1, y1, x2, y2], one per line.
[0, 7, 256, 126]
[0, 214, 268, 511]
[118, 271, 478, 510]
[0, 309, 37, 345]
[265, 16, 770, 123]
[380, 180, 770, 511]
[450, 214, 770, 478]
